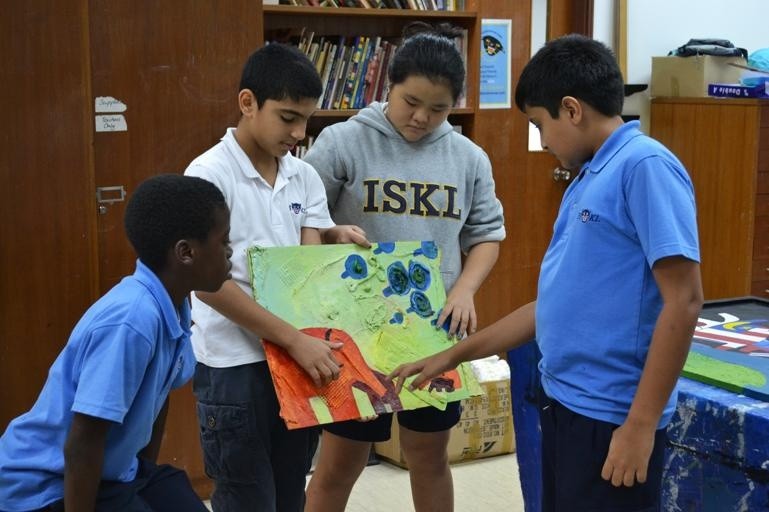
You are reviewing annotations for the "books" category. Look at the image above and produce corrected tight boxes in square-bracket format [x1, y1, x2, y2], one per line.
[260, 1, 474, 174]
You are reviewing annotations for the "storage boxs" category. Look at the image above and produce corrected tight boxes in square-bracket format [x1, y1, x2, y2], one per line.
[366, 378, 518, 474]
[648, 53, 752, 99]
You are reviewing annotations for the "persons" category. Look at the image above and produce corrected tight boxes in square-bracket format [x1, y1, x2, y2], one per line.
[385, 30, 707, 508]
[0, 171, 239, 511]
[289, 30, 510, 510]
[183, 39, 350, 510]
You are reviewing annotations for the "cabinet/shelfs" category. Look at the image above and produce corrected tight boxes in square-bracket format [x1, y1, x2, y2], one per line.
[649, 95, 769, 302]
[2, 1, 265, 502]
[264, 0, 480, 118]
